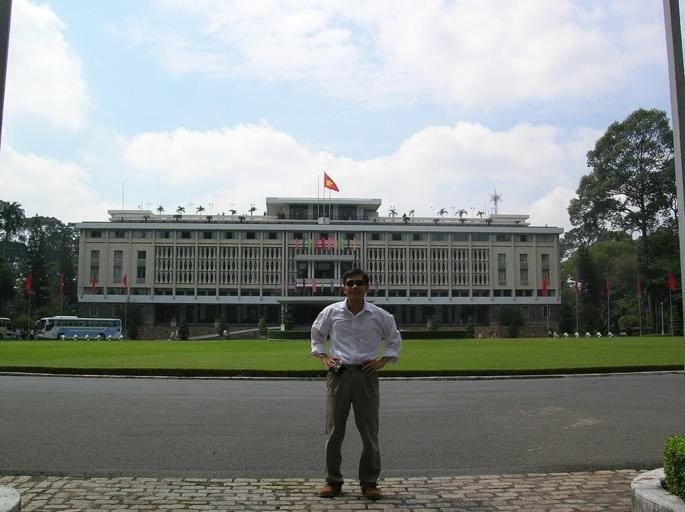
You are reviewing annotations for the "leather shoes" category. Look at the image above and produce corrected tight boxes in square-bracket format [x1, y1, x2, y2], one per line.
[365, 487, 382, 499]
[318, 486, 342, 498]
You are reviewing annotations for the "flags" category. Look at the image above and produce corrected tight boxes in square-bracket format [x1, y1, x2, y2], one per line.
[58, 274, 65, 294]
[605, 273, 610, 294]
[542, 275, 547, 295]
[282, 236, 380, 294]
[636, 273, 641, 296]
[575, 280, 579, 294]
[25, 273, 32, 295]
[91, 273, 96, 294]
[668, 272, 677, 291]
[324, 172, 339, 193]
[122, 275, 128, 296]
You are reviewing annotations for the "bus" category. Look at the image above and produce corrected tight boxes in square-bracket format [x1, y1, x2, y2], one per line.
[0, 318, 13, 339]
[34, 315, 124, 341]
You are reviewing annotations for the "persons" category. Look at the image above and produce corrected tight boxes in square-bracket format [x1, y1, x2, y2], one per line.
[309, 268, 403, 498]
[13, 327, 37, 340]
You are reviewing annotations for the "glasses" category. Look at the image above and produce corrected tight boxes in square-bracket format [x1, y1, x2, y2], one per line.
[346, 280, 365, 287]
